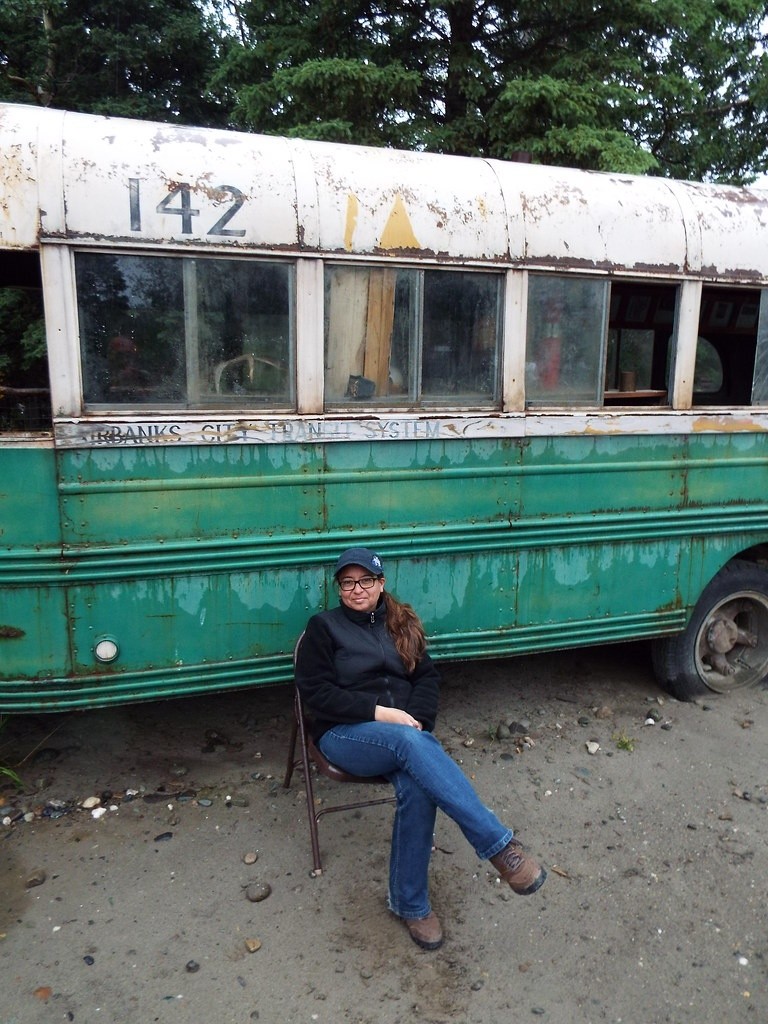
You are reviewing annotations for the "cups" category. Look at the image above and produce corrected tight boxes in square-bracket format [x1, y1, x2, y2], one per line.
[619, 371, 637, 391]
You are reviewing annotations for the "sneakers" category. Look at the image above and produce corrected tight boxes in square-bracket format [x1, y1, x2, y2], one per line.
[398, 909, 442, 950]
[488, 840, 545, 895]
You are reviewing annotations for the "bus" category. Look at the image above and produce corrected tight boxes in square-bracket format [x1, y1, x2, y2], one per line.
[0, 104, 768, 709]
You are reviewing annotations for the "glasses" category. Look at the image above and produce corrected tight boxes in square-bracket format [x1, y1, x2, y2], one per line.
[338, 578, 380, 591]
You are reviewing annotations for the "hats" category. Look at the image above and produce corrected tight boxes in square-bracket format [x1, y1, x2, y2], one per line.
[332, 548, 384, 574]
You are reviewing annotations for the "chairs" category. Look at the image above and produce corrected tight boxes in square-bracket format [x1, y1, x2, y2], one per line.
[282, 629, 439, 876]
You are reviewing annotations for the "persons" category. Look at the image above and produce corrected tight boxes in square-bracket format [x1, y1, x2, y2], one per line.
[295, 548, 547, 950]
[91, 337, 152, 402]
